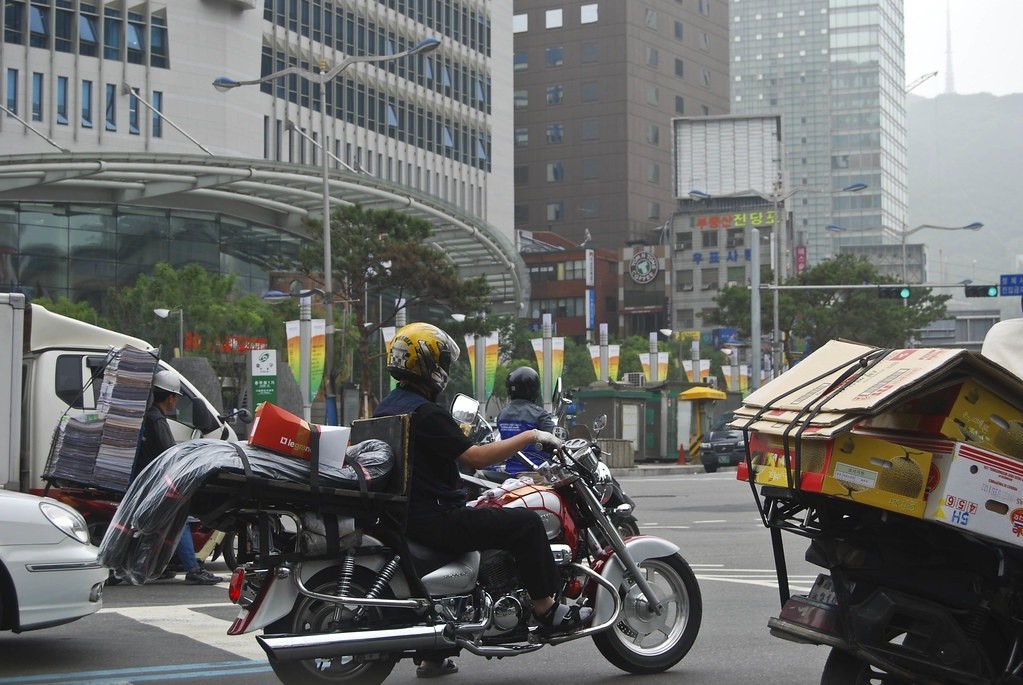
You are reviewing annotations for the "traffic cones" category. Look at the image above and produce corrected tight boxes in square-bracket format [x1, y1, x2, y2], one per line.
[677, 444, 687, 465]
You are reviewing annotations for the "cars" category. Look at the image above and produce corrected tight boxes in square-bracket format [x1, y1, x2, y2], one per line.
[0, 490, 111, 634]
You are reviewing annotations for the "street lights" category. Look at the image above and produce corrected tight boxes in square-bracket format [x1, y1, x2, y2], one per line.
[211, 39, 439, 427]
[824, 223, 984, 348]
[154, 308, 184, 358]
[686, 182, 868, 377]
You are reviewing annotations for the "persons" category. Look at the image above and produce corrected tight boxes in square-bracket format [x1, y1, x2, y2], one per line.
[496, 366, 554, 473]
[370, 322, 597, 678]
[135, 368, 225, 583]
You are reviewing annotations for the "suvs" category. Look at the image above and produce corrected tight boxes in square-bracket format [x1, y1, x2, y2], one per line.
[700, 410, 752, 473]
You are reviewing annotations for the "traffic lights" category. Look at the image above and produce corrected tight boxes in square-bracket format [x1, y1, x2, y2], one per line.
[879, 287, 909, 299]
[965, 285, 998, 298]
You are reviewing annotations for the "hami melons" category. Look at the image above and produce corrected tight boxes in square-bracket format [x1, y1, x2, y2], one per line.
[801, 440, 826, 473]
[842, 437, 855, 453]
[994, 420, 1023, 459]
[877, 447, 923, 498]
[967, 388, 979, 403]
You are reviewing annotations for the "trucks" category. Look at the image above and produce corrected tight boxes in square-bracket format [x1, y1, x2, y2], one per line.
[0, 293, 253, 489]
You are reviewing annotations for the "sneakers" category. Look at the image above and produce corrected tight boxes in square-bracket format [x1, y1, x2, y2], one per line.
[157, 569, 176, 579]
[185, 568, 223, 585]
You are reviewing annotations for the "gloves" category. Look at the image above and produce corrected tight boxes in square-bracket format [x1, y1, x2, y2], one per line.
[530, 429, 563, 452]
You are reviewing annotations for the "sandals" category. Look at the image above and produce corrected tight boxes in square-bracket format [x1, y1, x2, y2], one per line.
[531, 601, 596, 631]
[416, 657, 458, 678]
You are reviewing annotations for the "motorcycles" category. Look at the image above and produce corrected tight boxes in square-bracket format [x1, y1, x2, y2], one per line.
[760, 486, 1023, 685]
[29, 427, 280, 585]
[228, 377, 703, 685]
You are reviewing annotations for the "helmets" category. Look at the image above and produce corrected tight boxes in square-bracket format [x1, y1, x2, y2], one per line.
[506, 366, 540, 398]
[386, 322, 451, 394]
[154, 371, 183, 396]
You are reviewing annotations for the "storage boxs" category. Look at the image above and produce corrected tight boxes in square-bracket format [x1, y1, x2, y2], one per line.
[246, 401, 354, 469]
[736, 379, 1023, 550]
